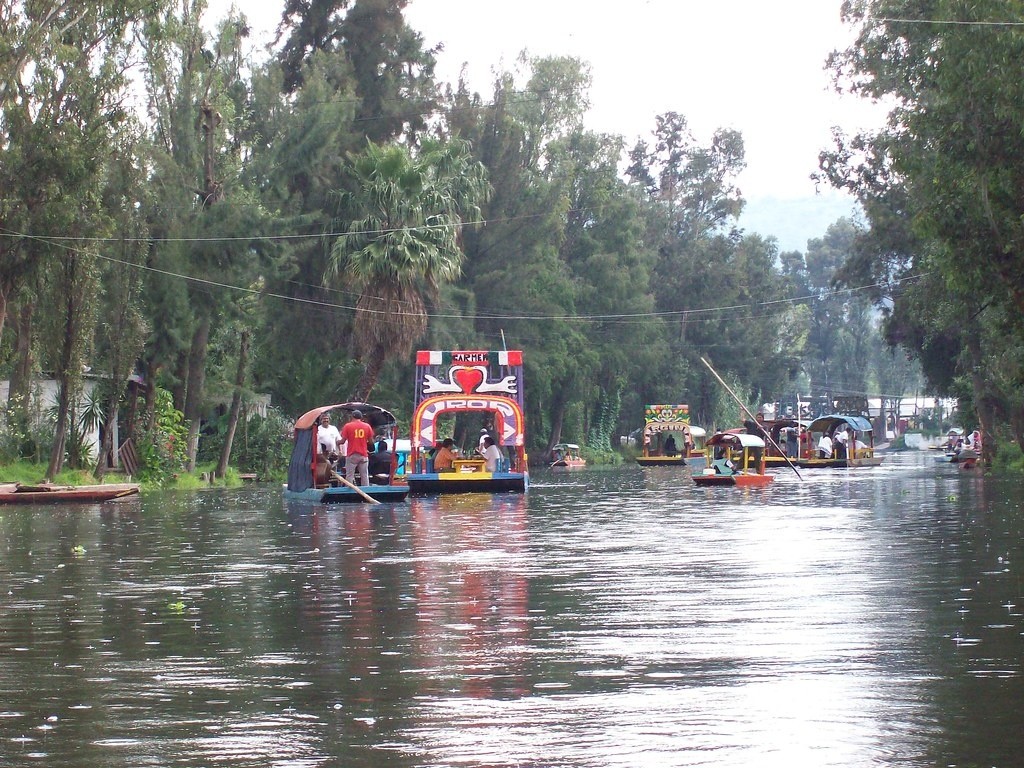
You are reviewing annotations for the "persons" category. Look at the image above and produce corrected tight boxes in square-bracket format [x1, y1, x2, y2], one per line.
[710, 450, 742, 476]
[736, 413, 768, 473]
[368, 429, 388, 474]
[337, 429, 348, 474]
[778, 421, 814, 459]
[436, 423, 442, 440]
[368, 441, 393, 485]
[316, 412, 343, 456]
[665, 434, 676, 457]
[473, 428, 505, 472]
[818, 432, 833, 458]
[832, 427, 871, 459]
[553, 449, 573, 462]
[336, 410, 374, 486]
[431, 438, 459, 472]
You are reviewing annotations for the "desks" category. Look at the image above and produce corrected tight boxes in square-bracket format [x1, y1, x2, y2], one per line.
[451, 459, 486, 473]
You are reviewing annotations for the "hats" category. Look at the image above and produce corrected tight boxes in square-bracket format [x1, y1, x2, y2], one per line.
[480, 429, 487, 432]
[351, 410, 362, 418]
[444, 438, 458, 444]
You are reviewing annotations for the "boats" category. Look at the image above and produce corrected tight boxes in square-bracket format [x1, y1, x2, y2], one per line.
[281, 402, 411, 504]
[722, 415, 887, 469]
[635, 422, 712, 467]
[549, 443, 587, 468]
[408, 350, 530, 492]
[928, 427, 982, 462]
[0, 484, 140, 502]
[692, 431, 775, 486]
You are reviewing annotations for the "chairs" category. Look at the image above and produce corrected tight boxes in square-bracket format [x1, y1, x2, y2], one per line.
[419, 456, 442, 474]
[495, 456, 510, 473]
